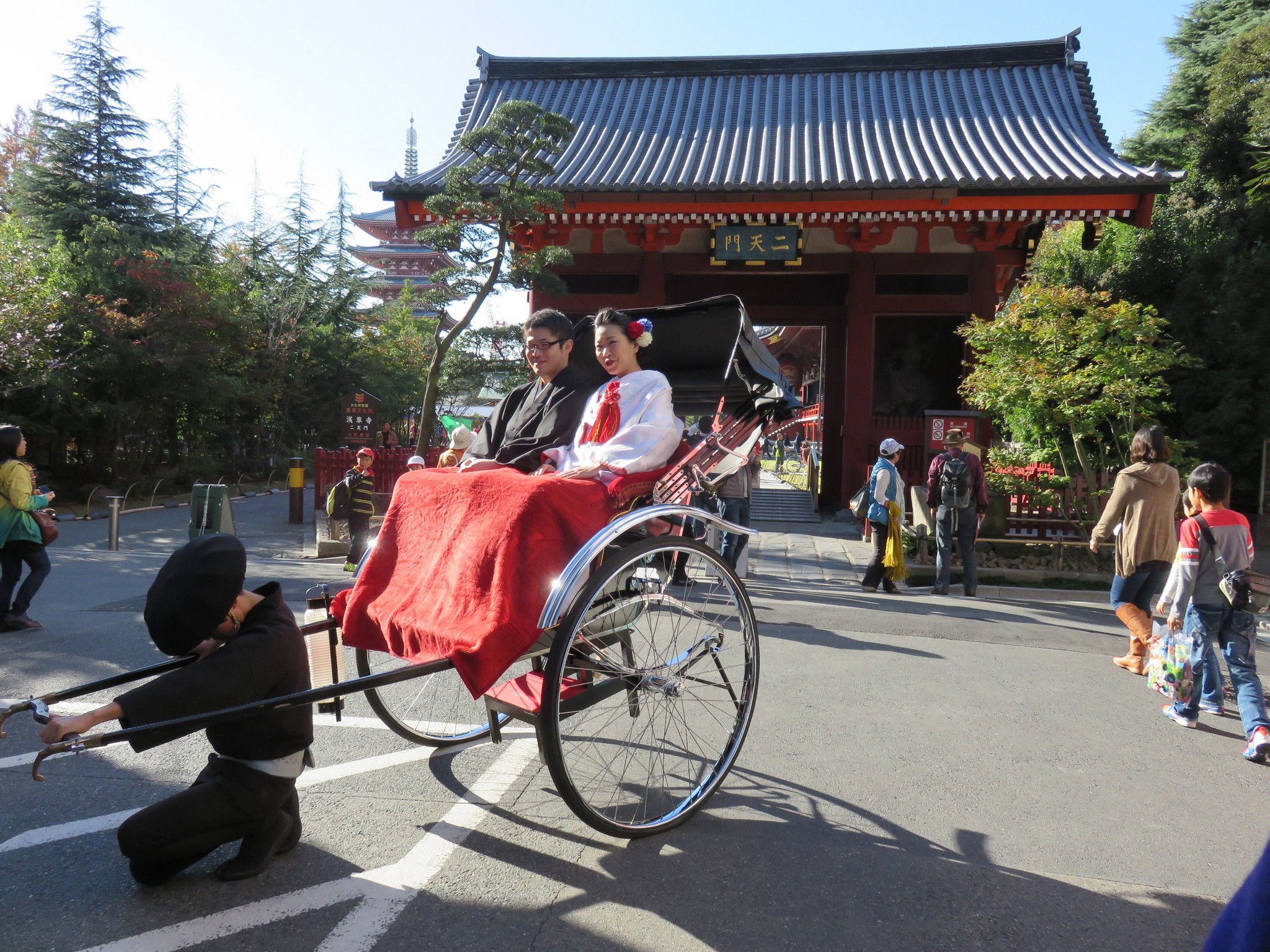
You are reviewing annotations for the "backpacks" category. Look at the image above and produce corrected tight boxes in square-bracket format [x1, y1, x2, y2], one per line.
[772, 445, 777, 456]
[940, 452, 974, 509]
[325, 479, 352, 521]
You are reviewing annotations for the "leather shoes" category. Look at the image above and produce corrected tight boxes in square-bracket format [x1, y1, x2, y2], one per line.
[0, 612, 44, 628]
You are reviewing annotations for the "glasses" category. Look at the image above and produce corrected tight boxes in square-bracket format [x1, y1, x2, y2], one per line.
[522, 338, 567, 351]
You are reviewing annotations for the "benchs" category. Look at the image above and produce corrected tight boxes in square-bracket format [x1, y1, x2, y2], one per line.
[606, 438, 692, 541]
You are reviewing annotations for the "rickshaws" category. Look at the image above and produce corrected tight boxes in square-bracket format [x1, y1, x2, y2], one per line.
[1, 291, 806, 838]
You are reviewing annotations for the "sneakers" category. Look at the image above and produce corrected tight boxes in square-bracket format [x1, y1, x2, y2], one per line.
[1242, 725, 1270, 762]
[1162, 703, 1196, 727]
[1198, 703, 1224, 716]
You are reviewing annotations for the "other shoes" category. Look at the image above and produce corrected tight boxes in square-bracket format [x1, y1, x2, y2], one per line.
[671, 578, 697, 587]
[343, 560, 356, 572]
[964, 588, 976, 597]
[929, 589, 948, 595]
[214, 785, 302, 883]
[886, 587, 901, 593]
[861, 585, 877, 592]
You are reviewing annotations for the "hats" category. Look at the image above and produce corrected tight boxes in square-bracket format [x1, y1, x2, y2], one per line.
[406, 456, 425, 468]
[942, 428, 967, 444]
[879, 438, 904, 456]
[356, 448, 374, 461]
[449, 426, 477, 450]
[143, 533, 247, 657]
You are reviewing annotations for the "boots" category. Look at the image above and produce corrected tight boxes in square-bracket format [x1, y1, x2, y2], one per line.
[1113, 602, 1155, 676]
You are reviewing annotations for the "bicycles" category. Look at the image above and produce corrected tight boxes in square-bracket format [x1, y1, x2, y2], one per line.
[785, 443, 821, 473]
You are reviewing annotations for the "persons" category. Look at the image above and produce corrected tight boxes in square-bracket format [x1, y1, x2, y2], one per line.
[860, 437, 907, 594]
[342, 448, 376, 572]
[404, 419, 483, 447]
[527, 306, 684, 489]
[373, 421, 399, 454]
[772, 433, 789, 473]
[714, 447, 763, 588]
[1156, 489, 1228, 716]
[1089, 424, 1180, 678]
[453, 309, 598, 474]
[0, 425, 56, 633]
[925, 428, 989, 596]
[801, 437, 811, 465]
[436, 426, 477, 469]
[407, 455, 426, 472]
[41, 532, 316, 887]
[783, 432, 793, 456]
[761, 434, 773, 460]
[671, 414, 718, 586]
[1167, 462, 1270, 762]
[794, 432, 801, 454]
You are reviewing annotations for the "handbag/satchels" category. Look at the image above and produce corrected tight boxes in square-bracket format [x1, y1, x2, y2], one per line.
[1146, 618, 1196, 705]
[29, 508, 59, 548]
[1218, 568, 1270, 615]
[849, 476, 871, 519]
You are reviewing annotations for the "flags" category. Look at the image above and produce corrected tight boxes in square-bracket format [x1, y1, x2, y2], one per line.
[440, 415, 473, 442]
